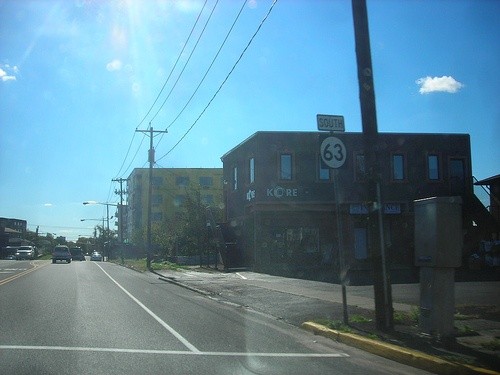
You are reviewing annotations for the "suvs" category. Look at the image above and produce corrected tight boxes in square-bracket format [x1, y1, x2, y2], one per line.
[15, 245, 34, 260]
[52, 244, 72, 264]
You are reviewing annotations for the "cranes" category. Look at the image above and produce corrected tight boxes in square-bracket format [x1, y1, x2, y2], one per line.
[70, 246, 83, 260]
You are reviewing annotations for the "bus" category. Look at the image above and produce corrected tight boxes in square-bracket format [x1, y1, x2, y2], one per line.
[0, 246, 19, 260]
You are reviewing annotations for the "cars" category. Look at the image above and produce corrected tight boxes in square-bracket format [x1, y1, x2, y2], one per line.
[90, 251, 102, 260]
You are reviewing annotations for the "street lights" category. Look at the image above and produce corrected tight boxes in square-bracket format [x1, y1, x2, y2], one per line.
[80, 218, 104, 261]
[83, 201, 110, 262]
